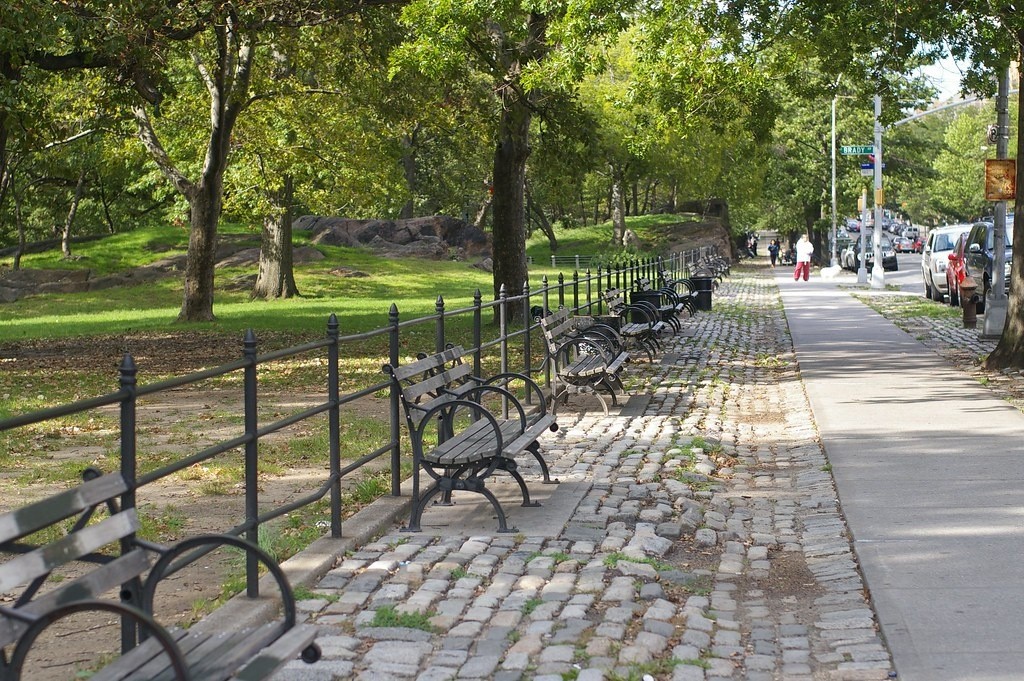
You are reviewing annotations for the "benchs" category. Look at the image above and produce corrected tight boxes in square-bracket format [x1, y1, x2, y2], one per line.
[0, 463, 323, 681]
[381, 253, 732, 532]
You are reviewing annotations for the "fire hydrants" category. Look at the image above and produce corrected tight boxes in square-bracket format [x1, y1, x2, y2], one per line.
[959, 276, 979, 329]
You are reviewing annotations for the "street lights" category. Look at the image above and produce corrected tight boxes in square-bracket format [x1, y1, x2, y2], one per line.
[858, 186, 867, 283]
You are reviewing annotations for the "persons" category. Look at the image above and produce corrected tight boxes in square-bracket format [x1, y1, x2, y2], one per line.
[794, 234, 814, 281]
[752, 241, 757, 256]
[768, 239, 779, 268]
[775, 238, 780, 246]
[787, 237, 796, 248]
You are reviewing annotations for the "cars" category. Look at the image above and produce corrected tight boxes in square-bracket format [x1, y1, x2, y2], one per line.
[843, 218, 928, 254]
[828, 230, 898, 275]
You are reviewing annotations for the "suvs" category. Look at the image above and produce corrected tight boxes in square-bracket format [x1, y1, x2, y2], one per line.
[921, 220, 1014, 314]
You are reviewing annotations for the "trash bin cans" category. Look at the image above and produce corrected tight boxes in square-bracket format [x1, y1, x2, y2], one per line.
[630, 291, 663, 338]
[689, 276, 713, 311]
[720, 256, 730, 275]
